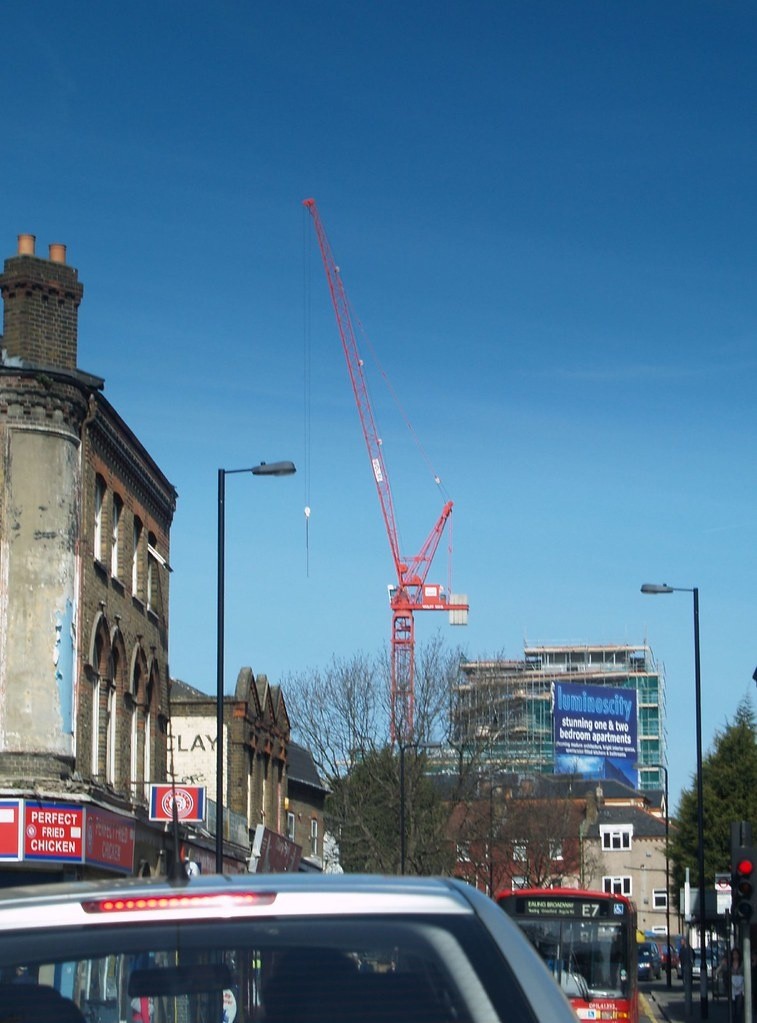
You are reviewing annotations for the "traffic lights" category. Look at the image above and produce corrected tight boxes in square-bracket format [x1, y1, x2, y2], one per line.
[729, 818, 757, 925]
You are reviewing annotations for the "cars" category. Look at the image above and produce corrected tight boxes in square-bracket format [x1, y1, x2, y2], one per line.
[654, 939, 680, 970]
[0, 870, 579, 1022]
[677, 947, 715, 980]
[638, 941, 662, 984]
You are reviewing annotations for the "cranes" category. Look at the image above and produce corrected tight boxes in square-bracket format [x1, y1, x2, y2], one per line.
[298, 194, 472, 748]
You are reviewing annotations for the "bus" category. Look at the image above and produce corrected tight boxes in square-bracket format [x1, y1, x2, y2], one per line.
[493, 887, 639, 1023]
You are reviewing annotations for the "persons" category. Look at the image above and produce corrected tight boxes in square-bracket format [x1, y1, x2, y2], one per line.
[133, 997, 151, 1023]
[714, 948, 744, 1022]
[679, 938, 697, 999]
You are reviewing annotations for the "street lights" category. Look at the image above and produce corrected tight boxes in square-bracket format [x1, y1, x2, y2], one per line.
[637, 581, 711, 1022]
[647, 762, 674, 988]
[212, 460, 298, 876]
